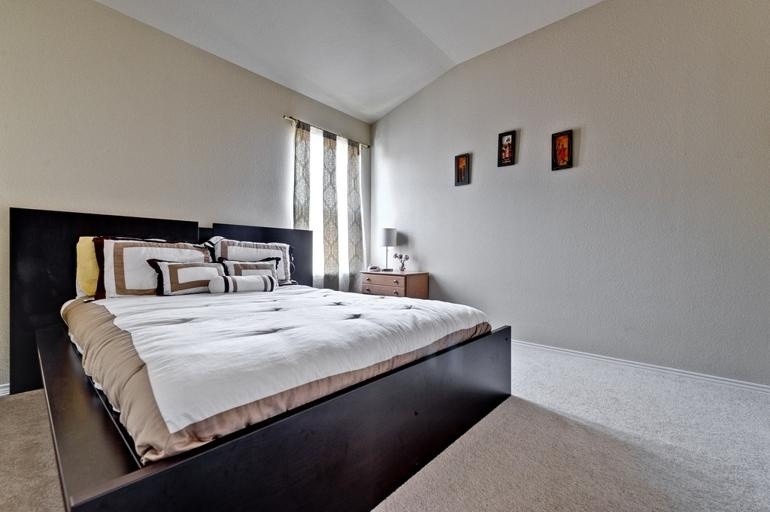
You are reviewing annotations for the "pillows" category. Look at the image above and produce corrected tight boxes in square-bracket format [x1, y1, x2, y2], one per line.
[75, 236, 292, 302]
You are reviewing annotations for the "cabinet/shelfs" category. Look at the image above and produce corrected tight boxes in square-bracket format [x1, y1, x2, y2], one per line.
[360, 271, 429, 300]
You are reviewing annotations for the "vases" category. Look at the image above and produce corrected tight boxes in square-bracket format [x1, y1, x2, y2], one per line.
[400, 267, 404, 271]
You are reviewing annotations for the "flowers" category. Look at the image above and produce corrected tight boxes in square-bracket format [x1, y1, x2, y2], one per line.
[394, 253, 409, 268]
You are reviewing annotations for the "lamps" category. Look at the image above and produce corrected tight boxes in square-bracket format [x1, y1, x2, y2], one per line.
[378, 228, 397, 272]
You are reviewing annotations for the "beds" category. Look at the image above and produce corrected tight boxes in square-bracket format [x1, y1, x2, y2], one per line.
[9, 207, 512, 512]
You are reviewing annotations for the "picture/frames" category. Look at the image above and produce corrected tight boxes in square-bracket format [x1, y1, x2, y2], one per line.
[551, 129, 572, 171]
[454, 153, 470, 186]
[498, 130, 516, 167]
